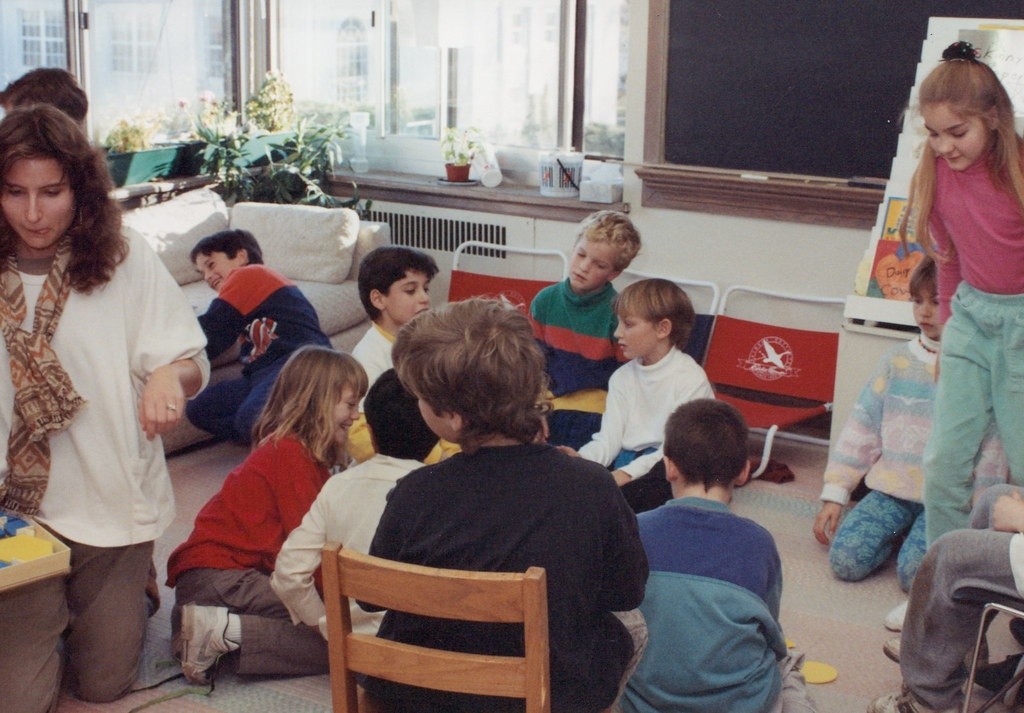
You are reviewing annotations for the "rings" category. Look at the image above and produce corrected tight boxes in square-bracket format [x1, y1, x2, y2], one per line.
[167, 404, 177, 411]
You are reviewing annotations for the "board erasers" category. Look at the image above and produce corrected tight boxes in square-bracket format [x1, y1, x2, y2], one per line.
[849, 175, 888, 190]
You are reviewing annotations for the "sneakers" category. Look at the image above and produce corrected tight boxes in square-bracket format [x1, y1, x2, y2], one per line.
[179, 604, 241, 685]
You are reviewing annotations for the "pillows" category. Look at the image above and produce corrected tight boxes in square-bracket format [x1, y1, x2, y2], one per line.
[122, 188, 225, 285]
[231, 202, 359, 285]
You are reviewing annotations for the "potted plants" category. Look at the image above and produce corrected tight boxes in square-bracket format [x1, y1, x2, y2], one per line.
[93, 68, 369, 215]
[438, 127, 480, 183]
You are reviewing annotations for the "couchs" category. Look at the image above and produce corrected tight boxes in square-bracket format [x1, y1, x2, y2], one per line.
[115, 207, 394, 451]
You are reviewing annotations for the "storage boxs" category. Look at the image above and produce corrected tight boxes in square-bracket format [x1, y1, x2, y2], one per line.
[0, 506, 71, 590]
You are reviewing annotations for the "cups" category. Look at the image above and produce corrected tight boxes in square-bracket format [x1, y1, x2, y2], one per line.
[473, 150, 502, 187]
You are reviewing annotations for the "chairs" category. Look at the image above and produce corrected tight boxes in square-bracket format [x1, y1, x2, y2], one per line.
[320, 540, 551, 713]
[446, 239, 852, 480]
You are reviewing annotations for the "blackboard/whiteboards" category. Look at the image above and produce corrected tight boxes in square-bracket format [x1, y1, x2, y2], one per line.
[634, 0, 1024, 231]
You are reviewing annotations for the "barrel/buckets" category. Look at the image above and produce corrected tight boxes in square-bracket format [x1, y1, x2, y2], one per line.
[538, 151, 587, 198]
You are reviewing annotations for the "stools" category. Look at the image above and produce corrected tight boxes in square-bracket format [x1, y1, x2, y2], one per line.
[954, 587, 1024, 713]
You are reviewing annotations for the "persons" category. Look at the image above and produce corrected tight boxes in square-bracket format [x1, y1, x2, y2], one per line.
[0, 66, 88, 126]
[165, 210, 817, 713]
[885, 41, 1024, 631]
[0, 105, 211, 713]
[865, 483, 1024, 713]
[813, 256, 1009, 594]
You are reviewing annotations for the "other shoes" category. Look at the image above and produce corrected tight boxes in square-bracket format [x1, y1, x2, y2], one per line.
[867, 689, 966, 713]
[883, 636, 902, 661]
[884, 599, 910, 630]
